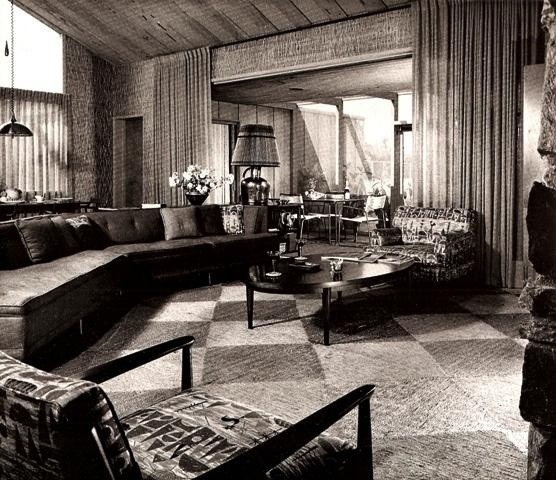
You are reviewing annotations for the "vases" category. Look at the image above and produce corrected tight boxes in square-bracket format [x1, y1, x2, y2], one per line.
[186, 193, 209, 206]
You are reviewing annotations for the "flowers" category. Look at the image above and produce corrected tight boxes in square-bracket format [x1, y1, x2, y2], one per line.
[169, 165, 234, 193]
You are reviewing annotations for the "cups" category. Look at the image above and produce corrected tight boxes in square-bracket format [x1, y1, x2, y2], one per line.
[330, 258, 343, 272]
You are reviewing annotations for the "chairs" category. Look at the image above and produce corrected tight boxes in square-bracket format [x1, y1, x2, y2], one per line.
[339, 195, 389, 247]
[0, 336, 376, 480]
[279, 193, 327, 239]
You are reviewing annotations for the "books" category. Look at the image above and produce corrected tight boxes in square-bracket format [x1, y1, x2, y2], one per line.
[321, 250, 387, 263]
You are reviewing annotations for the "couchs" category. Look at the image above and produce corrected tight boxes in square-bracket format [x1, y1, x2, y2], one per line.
[369, 206, 479, 306]
[1, 214, 126, 370]
[204, 206, 280, 282]
[89, 208, 214, 289]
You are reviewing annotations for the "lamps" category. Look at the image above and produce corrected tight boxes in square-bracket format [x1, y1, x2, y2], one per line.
[231, 125, 279, 206]
[1, 0, 34, 139]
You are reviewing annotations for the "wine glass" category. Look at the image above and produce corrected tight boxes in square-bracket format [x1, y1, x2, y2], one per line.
[265, 250, 282, 276]
[294, 239, 308, 260]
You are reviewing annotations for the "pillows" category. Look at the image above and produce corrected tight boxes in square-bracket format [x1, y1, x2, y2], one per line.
[160, 206, 200, 240]
[65, 214, 96, 249]
[196, 204, 226, 235]
[50, 216, 81, 254]
[219, 204, 245, 234]
[12, 216, 64, 263]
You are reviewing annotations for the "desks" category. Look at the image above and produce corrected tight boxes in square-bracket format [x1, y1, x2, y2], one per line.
[302, 199, 367, 245]
